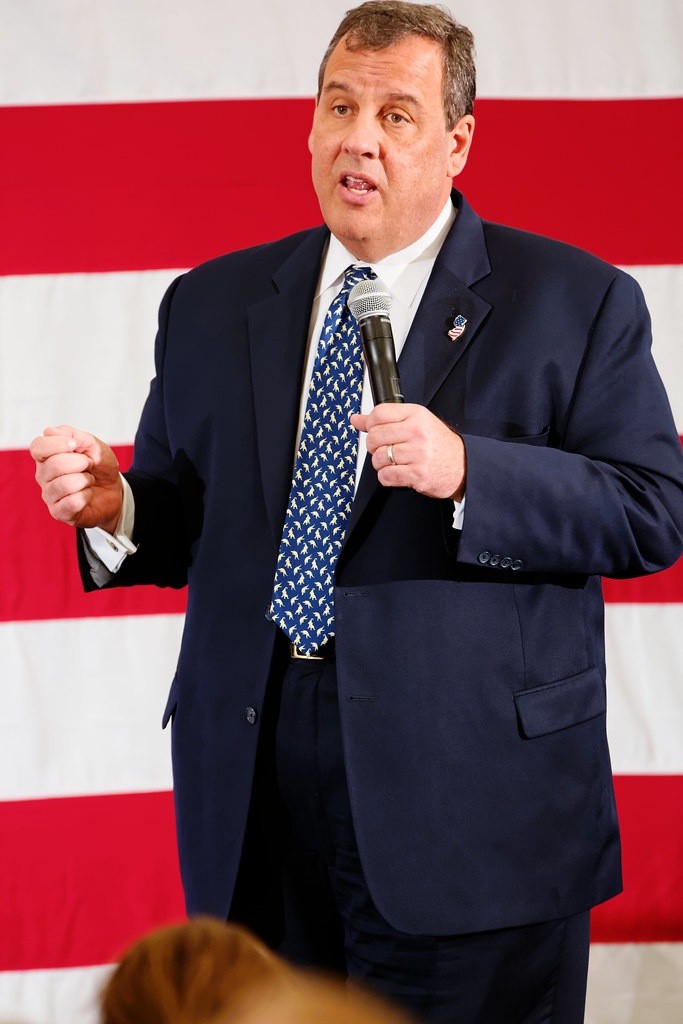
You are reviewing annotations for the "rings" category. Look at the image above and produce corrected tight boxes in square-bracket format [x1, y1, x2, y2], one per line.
[387, 445, 396, 464]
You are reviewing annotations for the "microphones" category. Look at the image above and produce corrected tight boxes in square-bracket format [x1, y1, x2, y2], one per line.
[347, 280, 405, 406]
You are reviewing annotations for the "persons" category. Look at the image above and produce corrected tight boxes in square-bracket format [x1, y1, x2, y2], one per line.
[27, 0, 683, 1024]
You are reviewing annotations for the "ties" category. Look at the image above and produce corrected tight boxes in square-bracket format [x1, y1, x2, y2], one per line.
[268, 265, 379, 656]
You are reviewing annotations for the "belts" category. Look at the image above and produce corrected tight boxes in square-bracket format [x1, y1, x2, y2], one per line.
[270, 623, 336, 662]
